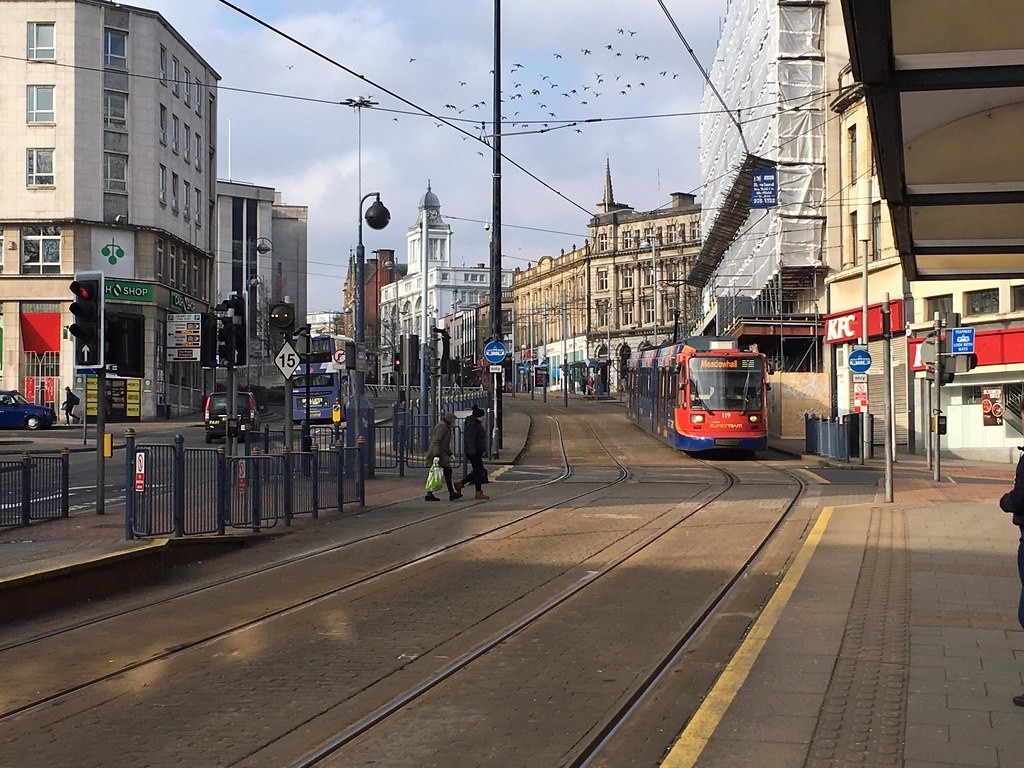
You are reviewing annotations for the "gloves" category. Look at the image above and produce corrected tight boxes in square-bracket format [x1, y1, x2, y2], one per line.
[433, 456, 440, 464]
[451, 454, 455, 460]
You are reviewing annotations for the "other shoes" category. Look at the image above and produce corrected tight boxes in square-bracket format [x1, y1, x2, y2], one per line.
[425, 495, 441, 501]
[449, 492, 464, 501]
[79, 417, 82, 425]
[64, 423, 70, 426]
[1012, 693, 1024, 706]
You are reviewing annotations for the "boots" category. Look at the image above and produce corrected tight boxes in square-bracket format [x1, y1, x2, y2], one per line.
[453, 480, 464, 494]
[474, 491, 489, 499]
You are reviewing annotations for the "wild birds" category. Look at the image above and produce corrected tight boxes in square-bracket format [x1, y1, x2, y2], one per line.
[409, 58, 416, 63]
[434, 27, 679, 159]
[285, 65, 295, 70]
[392, 117, 399, 122]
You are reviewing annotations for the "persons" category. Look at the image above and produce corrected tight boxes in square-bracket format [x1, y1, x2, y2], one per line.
[454, 405, 489, 498]
[63, 386, 82, 425]
[425, 412, 463, 501]
[580, 376, 594, 395]
[1000, 401, 1024, 707]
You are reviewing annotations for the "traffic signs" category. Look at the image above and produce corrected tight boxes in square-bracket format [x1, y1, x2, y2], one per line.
[166, 350, 201, 362]
[164, 313, 202, 349]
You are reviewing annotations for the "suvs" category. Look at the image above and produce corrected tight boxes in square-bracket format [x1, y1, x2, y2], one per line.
[204, 390, 265, 443]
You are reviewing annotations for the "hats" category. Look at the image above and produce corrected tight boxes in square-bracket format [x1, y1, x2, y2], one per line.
[471, 405, 485, 417]
[444, 412, 459, 421]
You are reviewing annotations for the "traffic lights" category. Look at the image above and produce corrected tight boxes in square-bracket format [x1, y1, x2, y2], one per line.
[219, 322, 236, 362]
[68, 271, 105, 369]
[394, 353, 400, 372]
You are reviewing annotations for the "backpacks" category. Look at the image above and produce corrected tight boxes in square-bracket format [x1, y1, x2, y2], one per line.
[72, 395, 79, 405]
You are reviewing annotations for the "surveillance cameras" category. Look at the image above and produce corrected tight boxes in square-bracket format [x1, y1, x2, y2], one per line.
[484, 223, 490, 231]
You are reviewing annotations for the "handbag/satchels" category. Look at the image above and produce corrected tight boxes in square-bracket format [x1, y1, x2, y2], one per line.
[426, 459, 443, 492]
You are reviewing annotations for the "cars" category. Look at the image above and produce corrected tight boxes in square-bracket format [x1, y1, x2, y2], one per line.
[0, 390, 56, 431]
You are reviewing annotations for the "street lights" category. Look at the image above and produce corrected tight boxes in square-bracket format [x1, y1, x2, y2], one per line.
[244, 233, 275, 479]
[354, 191, 392, 501]
[639, 229, 657, 346]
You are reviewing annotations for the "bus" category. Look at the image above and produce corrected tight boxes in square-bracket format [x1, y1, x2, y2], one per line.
[625, 333, 775, 452]
[290, 332, 379, 426]
